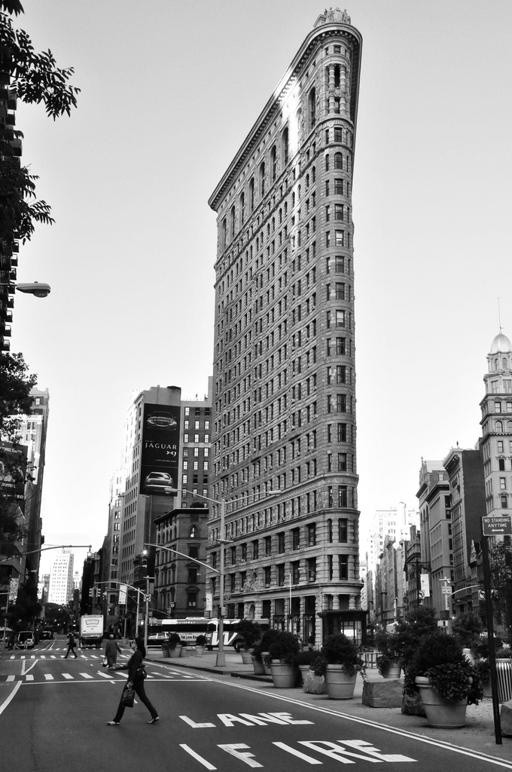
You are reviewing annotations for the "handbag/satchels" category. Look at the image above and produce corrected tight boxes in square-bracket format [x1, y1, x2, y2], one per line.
[122, 690, 134, 706]
[136, 667, 147, 680]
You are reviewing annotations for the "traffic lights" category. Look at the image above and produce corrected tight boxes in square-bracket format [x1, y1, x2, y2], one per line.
[89, 586, 101, 599]
[87, 552, 95, 560]
[134, 550, 148, 577]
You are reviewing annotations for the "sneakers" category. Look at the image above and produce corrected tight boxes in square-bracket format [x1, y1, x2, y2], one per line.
[107, 721, 120, 725]
[148, 716, 159, 724]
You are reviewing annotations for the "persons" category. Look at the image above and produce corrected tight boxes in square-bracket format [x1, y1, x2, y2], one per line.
[104, 637, 160, 726]
[64, 634, 78, 658]
[104, 634, 121, 668]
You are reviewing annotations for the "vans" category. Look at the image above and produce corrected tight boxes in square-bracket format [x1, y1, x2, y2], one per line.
[17, 630, 36, 648]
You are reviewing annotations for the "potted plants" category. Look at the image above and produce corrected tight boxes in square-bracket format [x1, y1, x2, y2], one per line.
[233, 608, 502, 729]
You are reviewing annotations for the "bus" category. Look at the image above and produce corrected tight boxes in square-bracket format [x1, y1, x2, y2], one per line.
[136, 618, 270, 650]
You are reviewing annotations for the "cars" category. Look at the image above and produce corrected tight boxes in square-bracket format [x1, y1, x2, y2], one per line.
[146, 411, 177, 432]
[144, 472, 174, 494]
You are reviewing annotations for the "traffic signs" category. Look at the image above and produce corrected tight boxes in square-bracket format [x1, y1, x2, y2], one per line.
[482, 515, 512, 536]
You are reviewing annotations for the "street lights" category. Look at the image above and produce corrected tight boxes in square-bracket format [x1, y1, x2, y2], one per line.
[163, 487, 281, 667]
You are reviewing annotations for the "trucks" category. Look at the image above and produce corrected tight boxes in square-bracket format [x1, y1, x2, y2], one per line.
[79, 614, 105, 648]
